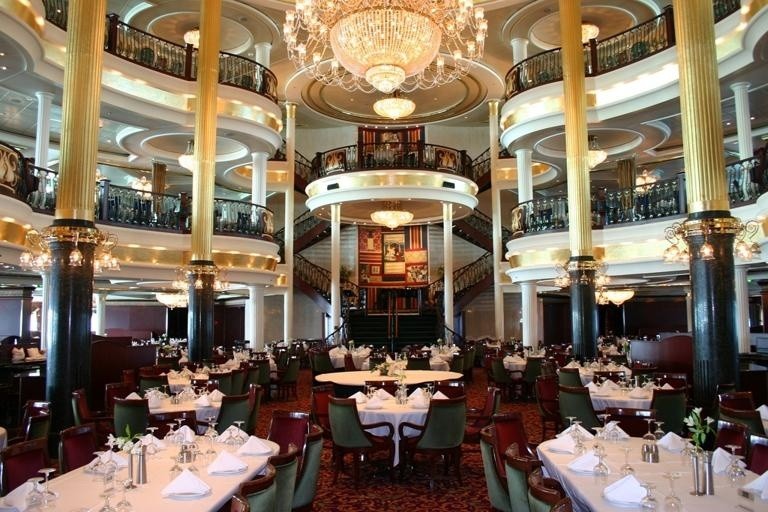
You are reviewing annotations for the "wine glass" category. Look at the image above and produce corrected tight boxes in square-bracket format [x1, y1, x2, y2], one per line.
[27, 415, 246, 511]
[143, 385, 212, 406]
[568, 357, 662, 399]
[565, 414, 746, 510]
[359, 382, 435, 407]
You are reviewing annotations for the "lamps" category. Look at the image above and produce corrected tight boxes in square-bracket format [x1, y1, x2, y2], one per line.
[582, 24, 599, 43]
[553, 275, 633, 308]
[155, 270, 226, 310]
[178, 141, 194, 172]
[183, 27, 200, 47]
[588, 140, 608, 171]
[284, 1, 489, 97]
[373, 98, 416, 120]
[20, 229, 121, 273]
[370, 211, 414, 228]
[664, 232, 763, 263]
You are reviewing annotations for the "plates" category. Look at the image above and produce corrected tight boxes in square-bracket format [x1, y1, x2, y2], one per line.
[168, 446, 274, 501]
[548, 442, 646, 508]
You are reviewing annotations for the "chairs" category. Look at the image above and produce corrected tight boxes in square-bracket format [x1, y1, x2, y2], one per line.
[0, 336, 768, 512]
